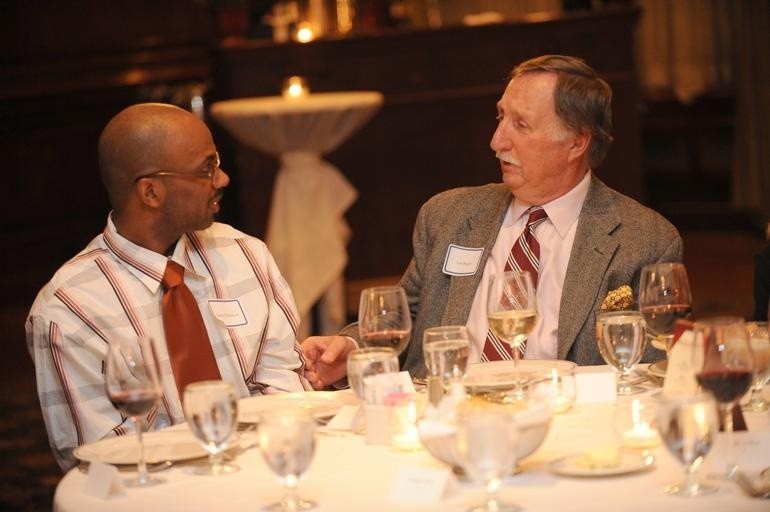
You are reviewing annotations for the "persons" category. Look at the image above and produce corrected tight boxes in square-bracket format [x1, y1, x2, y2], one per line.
[24, 102, 315, 481]
[302, 50, 688, 392]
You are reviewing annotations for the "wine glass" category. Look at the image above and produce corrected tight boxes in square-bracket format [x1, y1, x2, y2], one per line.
[695, 316, 755, 445]
[104, 332, 166, 487]
[652, 390, 730, 502]
[249, 414, 317, 512]
[484, 271, 539, 383]
[185, 381, 241, 477]
[596, 312, 646, 397]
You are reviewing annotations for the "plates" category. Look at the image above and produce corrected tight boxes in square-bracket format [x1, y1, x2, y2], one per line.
[546, 453, 655, 477]
[234, 393, 341, 422]
[464, 358, 580, 394]
[417, 416, 548, 479]
[73, 430, 242, 466]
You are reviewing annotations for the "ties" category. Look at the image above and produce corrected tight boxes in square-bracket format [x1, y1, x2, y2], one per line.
[157, 258, 225, 421]
[478, 205, 550, 366]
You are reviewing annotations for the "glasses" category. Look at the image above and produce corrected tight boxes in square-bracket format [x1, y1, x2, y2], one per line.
[133, 150, 221, 184]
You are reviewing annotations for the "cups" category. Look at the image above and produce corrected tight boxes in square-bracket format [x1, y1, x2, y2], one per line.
[357, 286, 412, 356]
[421, 324, 470, 388]
[345, 346, 417, 454]
[637, 264, 692, 352]
